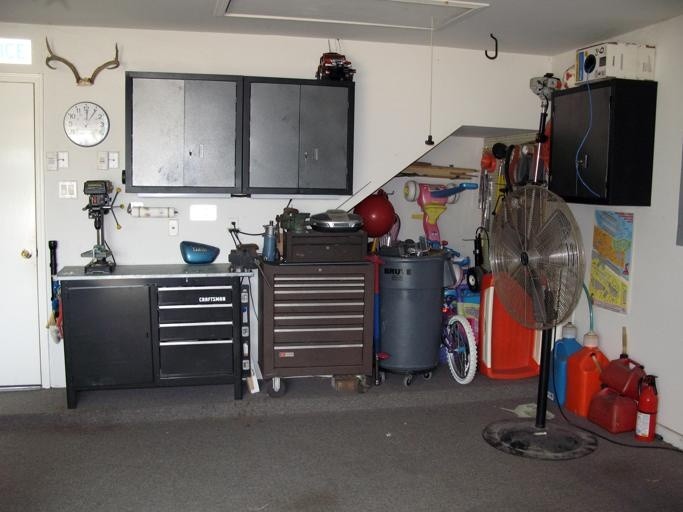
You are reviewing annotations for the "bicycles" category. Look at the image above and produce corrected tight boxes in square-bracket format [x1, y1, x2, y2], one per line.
[441, 288, 480, 385]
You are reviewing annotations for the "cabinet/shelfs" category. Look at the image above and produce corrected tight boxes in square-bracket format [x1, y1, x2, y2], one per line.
[546, 78, 658, 206]
[257, 260, 382, 398]
[56, 279, 246, 407]
[125, 70, 240, 197]
[240, 75, 355, 197]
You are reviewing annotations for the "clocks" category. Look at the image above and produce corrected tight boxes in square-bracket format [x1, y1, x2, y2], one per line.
[63, 101, 111, 146]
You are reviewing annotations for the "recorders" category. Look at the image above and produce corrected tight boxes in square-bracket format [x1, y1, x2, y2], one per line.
[310, 209, 365, 232]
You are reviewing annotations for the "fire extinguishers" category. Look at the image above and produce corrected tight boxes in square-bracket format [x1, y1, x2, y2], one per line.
[633, 374, 658, 443]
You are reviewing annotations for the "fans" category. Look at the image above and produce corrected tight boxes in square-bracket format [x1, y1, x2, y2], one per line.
[474, 185, 608, 461]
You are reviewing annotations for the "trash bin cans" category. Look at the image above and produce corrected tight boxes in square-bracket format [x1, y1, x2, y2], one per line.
[372, 255, 444, 388]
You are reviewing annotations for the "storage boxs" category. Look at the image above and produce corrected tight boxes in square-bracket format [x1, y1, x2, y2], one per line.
[572, 39, 656, 86]
